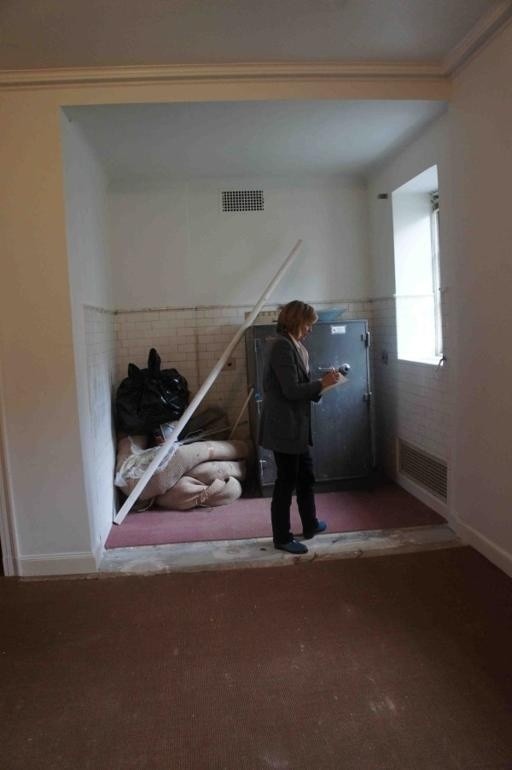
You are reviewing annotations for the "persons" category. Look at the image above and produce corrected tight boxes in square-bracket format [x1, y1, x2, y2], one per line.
[257, 300, 339, 555]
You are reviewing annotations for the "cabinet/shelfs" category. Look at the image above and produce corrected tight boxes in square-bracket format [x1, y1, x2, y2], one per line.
[243, 319, 378, 498]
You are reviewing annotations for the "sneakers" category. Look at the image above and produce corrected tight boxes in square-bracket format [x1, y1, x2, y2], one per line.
[274, 539, 308, 554]
[304, 521, 326, 539]
